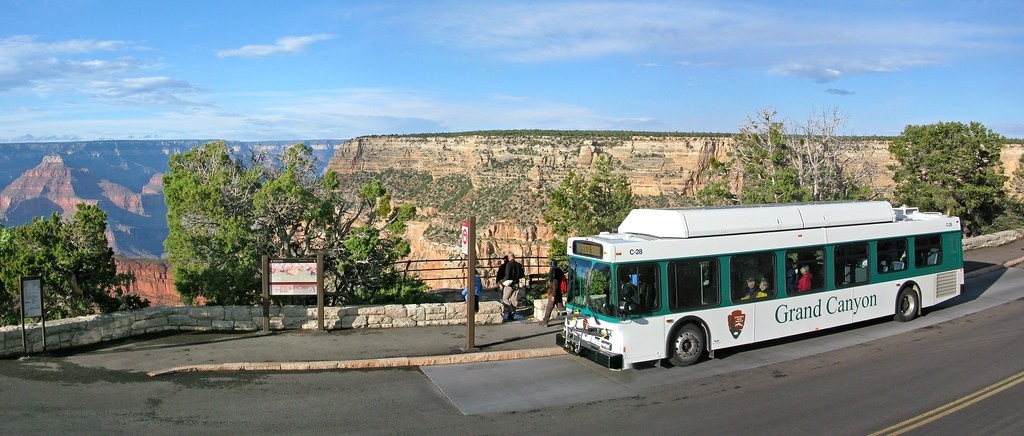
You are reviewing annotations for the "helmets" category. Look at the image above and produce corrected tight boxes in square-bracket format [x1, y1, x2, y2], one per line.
[550, 261, 557, 267]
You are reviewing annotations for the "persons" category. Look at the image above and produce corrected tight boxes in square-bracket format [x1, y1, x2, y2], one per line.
[752, 277, 772, 298]
[499, 253, 524, 322]
[798, 265, 813, 293]
[495, 256, 509, 297]
[787, 258, 796, 295]
[742, 276, 759, 299]
[856, 253, 868, 268]
[620, 275, 644, 312]
[461, 268, 483, 314]
[539, 261, 568, 327]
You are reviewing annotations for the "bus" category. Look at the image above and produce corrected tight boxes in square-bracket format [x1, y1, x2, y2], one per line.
[556, 205, 966, 369]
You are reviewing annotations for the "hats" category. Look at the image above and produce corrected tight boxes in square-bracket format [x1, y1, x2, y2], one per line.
[757, 276, 768, 281]
[745, 276, 755, 282]
[801, 265, 809, 271]
[502, 256, 508, 259]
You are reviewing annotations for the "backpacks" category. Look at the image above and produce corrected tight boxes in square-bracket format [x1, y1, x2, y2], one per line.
[561, 276, 567, 294]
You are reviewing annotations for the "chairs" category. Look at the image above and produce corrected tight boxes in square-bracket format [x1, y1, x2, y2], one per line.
[636, 274, 655, 308]
[889, 261, 904, 271]
[922, 251, 938, 265]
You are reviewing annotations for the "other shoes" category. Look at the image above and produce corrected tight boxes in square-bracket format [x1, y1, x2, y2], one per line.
[511, 311, 515, 314]
[539, 321, 548, 327]
[507, 316, 514, 321]
